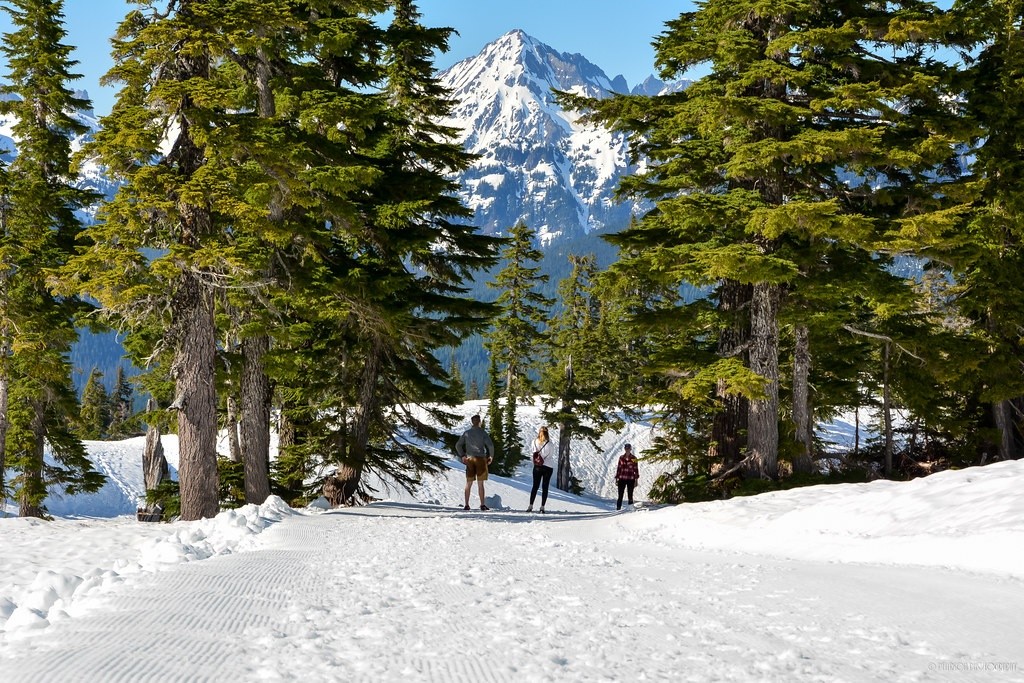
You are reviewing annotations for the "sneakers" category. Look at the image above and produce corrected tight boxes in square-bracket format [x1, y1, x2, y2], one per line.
[539, 507, 545, 514]
[481, 505, 489, 511]
[526, 505, 532, 512]
[464, 506, 471, 510]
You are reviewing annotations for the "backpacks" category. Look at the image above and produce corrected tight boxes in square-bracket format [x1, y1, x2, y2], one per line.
[533, 438, 549, 466]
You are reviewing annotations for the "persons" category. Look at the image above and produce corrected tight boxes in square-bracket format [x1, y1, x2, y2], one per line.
[454, 413, 494, 510]
[525, 426, 555, 515]
[614, 444, 640, 512]
